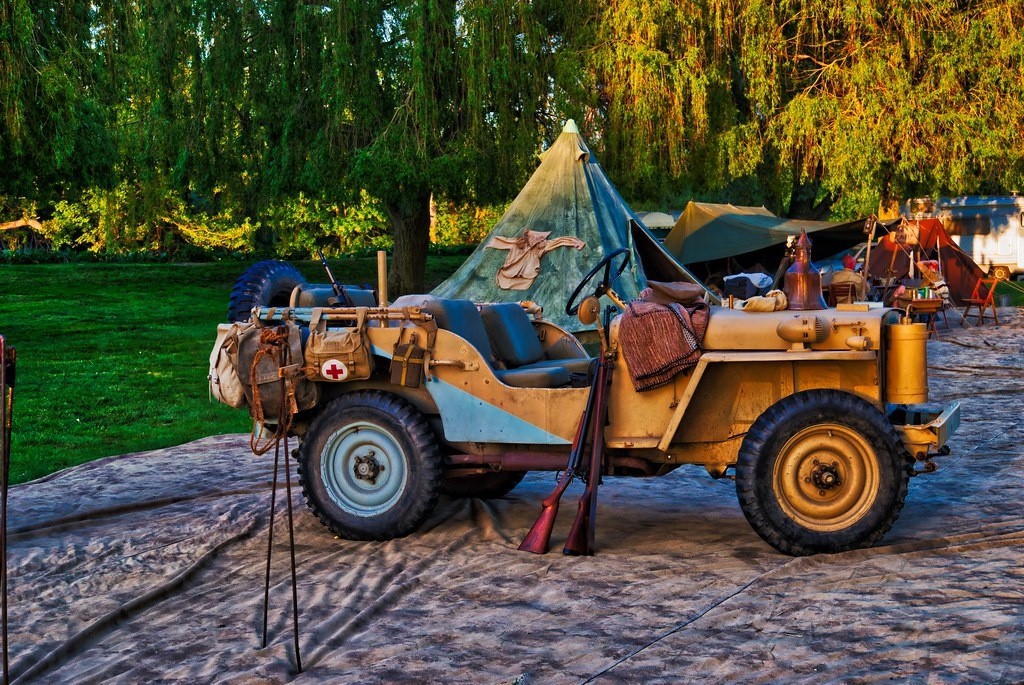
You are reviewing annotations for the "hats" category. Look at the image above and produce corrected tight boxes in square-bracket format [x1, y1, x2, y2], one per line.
[642, 280, 702, 304]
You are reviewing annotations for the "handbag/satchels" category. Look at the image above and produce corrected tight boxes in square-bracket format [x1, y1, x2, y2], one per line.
[306, 307, 374, 383]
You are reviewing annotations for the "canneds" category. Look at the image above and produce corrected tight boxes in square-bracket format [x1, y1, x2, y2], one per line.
[928, 290, 934, 299]
[923, 287, 930, 299]
[911, 290, 918, 299]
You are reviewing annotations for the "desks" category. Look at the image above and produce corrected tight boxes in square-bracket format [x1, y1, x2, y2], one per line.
[899, 297, 944, 341]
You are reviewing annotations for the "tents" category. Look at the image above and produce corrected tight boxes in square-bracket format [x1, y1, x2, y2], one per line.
[853, 220, 989, 303]
[663, 200, 902, 283]
[426, 118, 724, 334]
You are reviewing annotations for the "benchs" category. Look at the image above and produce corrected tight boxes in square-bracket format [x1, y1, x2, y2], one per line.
[300, 289, 376, 326]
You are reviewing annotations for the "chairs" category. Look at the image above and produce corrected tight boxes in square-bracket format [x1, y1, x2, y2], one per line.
[828, 283, 859, 305]
[426, 297, 571, 385]
[959, 277, 999, 327]
[482, 303, 601, 375]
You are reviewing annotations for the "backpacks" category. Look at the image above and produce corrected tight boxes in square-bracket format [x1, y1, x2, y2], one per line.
[207, 305, 260, 410]
[236, 307, 321, 455]
